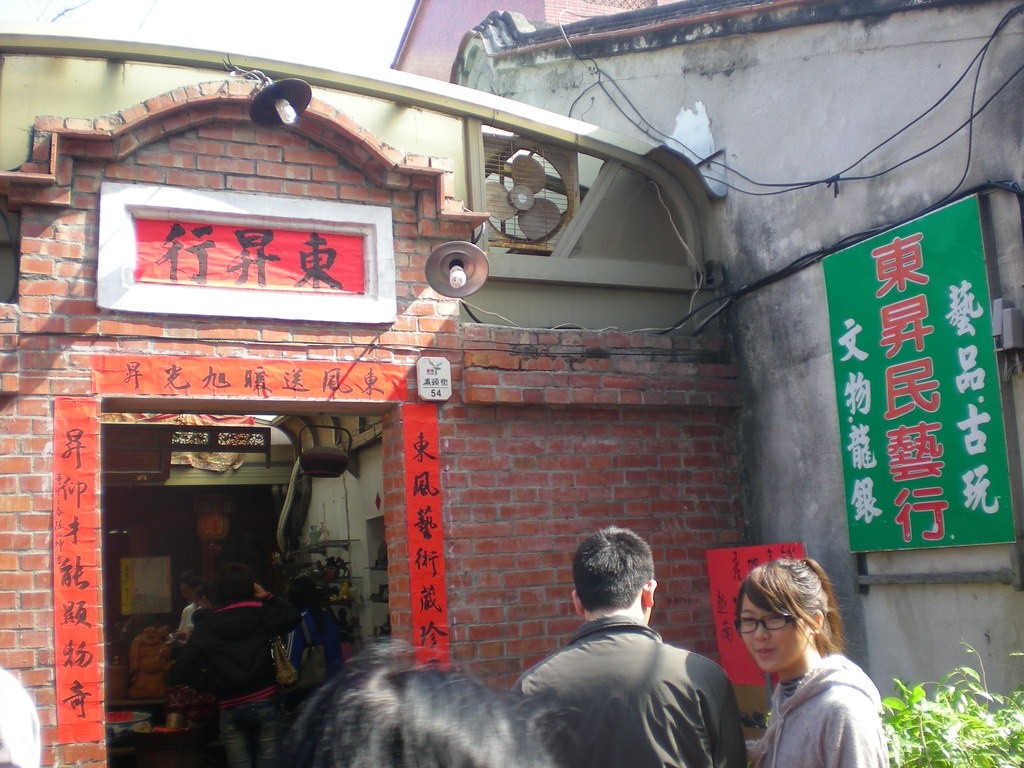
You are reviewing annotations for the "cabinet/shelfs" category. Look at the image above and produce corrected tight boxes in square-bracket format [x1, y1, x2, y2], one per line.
[292, 538, 361, 642]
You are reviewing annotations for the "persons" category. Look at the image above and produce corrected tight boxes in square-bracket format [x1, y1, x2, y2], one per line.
[504, 525, 749, 768]
[735, 556, 890, 768]
[128, 627, 172, 699]
[282, 637, 583, 768]
[0, 667, 41, 768]
[162, 558, 345, 768]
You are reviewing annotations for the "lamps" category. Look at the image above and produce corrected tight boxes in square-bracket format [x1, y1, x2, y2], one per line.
[425, 239, 489, 298]
[249, 77, 312, 129]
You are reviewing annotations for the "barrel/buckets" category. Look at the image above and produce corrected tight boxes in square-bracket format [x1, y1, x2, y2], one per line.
[299, 425, 352, 478]
[105, 712, 152, 753]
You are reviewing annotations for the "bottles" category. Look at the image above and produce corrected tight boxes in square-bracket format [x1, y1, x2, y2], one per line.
[309, 522, 329, 544]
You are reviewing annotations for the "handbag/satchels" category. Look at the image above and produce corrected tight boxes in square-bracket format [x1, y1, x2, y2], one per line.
[261, 610, 298, 687]
[293, 615, 328, 688]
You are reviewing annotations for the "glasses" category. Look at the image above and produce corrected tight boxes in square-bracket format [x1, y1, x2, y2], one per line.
[733, 612, 792, 634]
